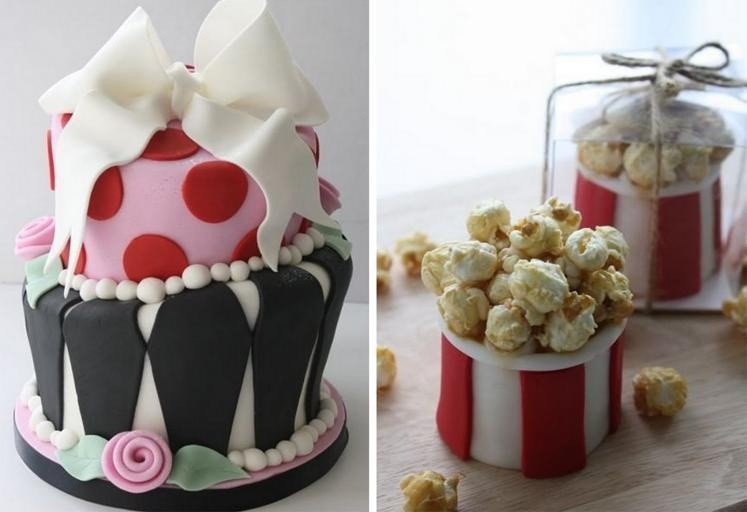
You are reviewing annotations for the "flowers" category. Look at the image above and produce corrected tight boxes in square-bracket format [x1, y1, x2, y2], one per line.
[14, 217, 64, 310]
[56, 428, 251, 494]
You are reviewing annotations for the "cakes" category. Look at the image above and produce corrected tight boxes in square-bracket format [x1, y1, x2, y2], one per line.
[418, 193, 633, 481]
[12, 0, 354, 511]
[572, 98, 737, 303]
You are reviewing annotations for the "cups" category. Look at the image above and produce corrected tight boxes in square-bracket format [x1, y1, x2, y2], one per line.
[436, 312, 628, 474]
[573, 150, 728, 310]
